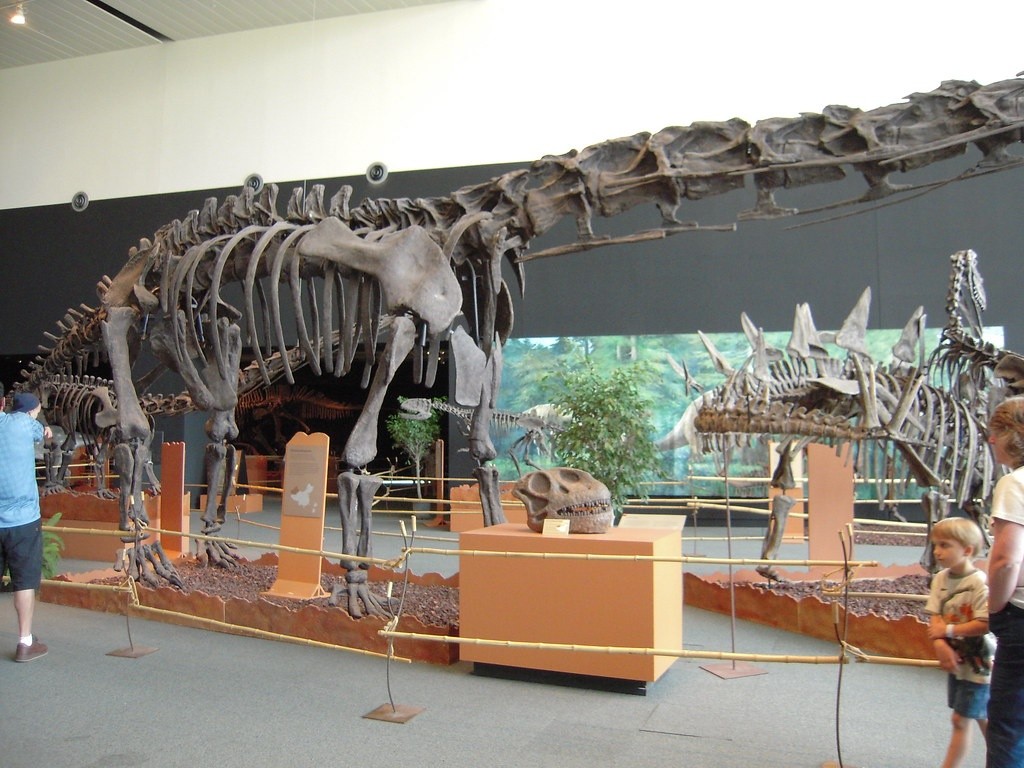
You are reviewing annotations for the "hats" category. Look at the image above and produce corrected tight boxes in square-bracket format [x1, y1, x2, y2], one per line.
[11, 393, 39, 413]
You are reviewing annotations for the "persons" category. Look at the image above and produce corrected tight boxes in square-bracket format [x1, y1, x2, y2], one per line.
[986, 396, 1024, 768]
[924, 517, 998, 768]
[0, 383, 47, 662]
[9, 394, 53, 438]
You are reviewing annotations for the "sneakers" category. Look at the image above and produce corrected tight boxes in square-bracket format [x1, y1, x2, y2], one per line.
[15, 635, 48, 662]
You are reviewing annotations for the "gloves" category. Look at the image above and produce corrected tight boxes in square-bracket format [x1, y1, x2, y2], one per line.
[989, 609, 1009, 637]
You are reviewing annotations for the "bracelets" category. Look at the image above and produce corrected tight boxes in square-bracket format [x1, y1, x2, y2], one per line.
[946, 624, 955, 640]
